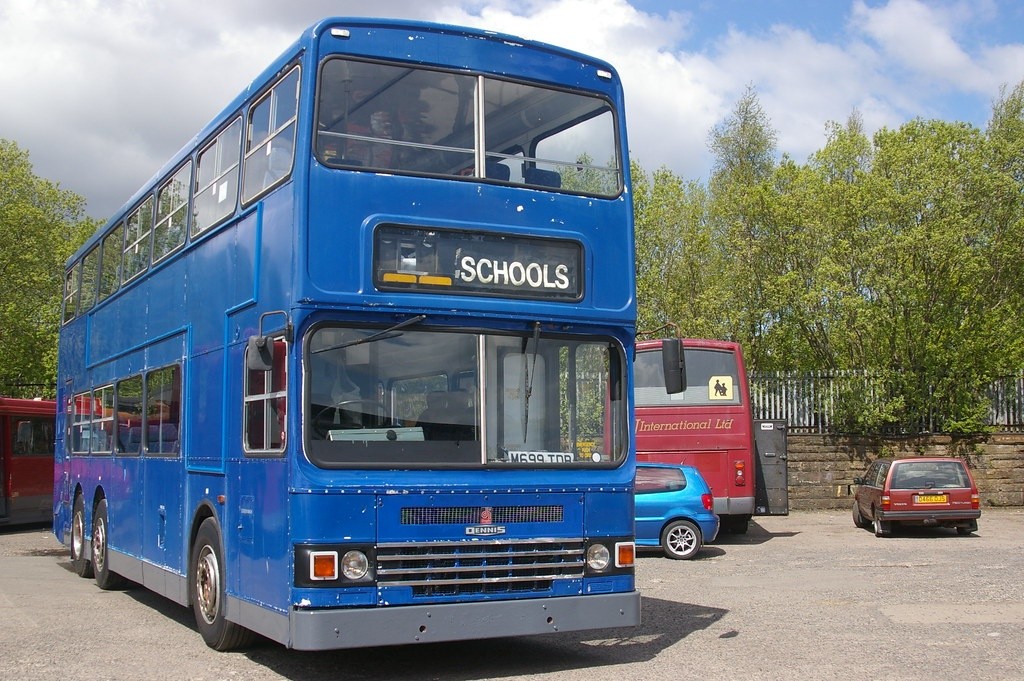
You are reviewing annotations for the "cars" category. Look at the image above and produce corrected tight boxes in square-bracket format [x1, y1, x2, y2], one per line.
[634, 462, 719, 560]
[852, 456, 981, 537]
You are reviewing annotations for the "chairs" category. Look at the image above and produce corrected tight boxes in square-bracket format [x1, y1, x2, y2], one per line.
[416, 390, 475, 441]
[111, 423, 179, 453]
[469, 162, 510, 182]
[327, 158, 363, 166]
[525, 167, 562, 188]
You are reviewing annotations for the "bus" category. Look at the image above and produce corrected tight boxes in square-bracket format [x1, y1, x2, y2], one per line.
[603, 339, 757, 535]
[0, 397, 56, 526]
[53, 16, 689, 653]
